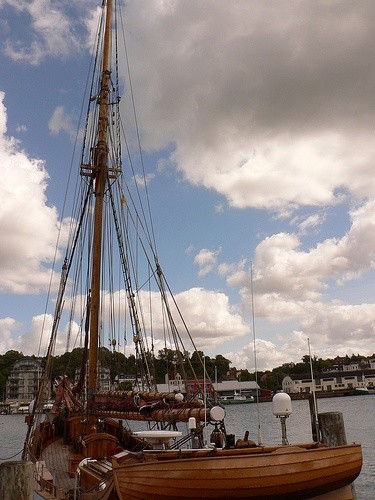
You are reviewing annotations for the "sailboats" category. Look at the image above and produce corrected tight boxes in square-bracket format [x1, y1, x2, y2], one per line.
[22, 0, 363, 500]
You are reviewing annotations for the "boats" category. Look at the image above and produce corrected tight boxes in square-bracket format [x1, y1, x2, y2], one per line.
[1, 373, 374, 413]
